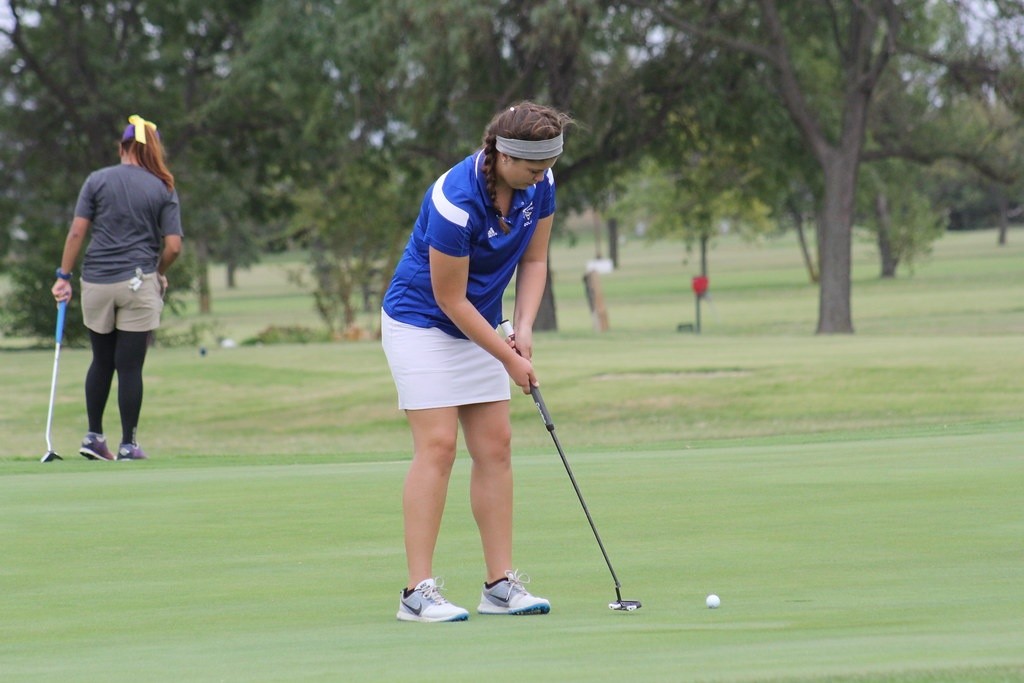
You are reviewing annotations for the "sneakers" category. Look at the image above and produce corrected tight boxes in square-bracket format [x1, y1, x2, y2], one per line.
[396, 577, 469, 622]
[80, 432, 117, 461]
[118, 442, 148, 461]
[476, 569, 551, 615]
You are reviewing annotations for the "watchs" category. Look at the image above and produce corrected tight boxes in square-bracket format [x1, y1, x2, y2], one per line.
[54, 268, 73, 279]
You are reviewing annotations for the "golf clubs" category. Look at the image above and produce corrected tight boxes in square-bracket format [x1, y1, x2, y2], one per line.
[41, 299, 65, 464]
[500, 317, 644, 611]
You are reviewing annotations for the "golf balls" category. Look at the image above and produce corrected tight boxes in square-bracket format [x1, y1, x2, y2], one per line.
[707, 594, 720, 608]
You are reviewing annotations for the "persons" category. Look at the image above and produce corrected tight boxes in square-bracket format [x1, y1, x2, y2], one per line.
[51, 113, 185, 460]
[380, 103, 564, 627]
